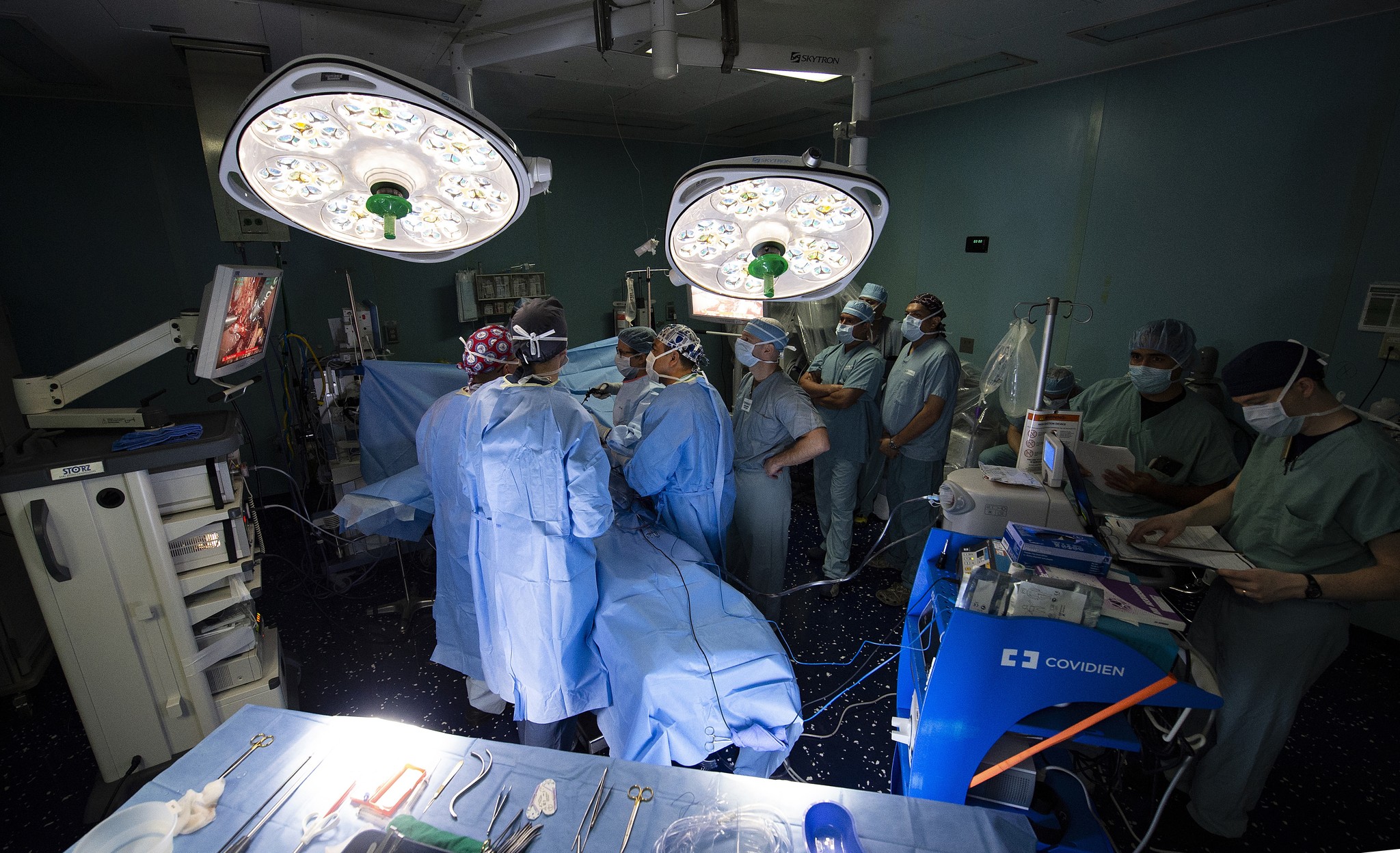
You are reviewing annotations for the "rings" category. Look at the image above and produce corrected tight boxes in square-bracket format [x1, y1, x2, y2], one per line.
[1243, 589, 1246, 597]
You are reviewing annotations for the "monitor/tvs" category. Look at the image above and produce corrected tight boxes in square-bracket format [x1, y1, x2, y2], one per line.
[194, 262, 284, 380]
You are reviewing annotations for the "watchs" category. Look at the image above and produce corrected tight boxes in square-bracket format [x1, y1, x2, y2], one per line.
[888, 438, 899, 454]
[1302, 572, 1324, 602]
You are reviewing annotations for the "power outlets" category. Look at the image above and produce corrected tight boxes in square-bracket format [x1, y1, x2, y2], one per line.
[1378, 332, 1400, 361]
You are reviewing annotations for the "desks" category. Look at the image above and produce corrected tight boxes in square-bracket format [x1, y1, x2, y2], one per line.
[59, 702, 1038, 853]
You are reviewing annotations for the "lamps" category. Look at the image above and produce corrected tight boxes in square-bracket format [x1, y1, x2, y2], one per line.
[665, 146, 890, 303]
[217, 51, 536, 265]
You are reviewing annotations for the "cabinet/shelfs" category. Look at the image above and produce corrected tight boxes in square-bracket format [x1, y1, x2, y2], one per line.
[2, 411, 288, 781]
[476, 272, 547, 315]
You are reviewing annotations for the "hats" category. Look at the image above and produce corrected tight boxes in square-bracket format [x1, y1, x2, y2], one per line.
[908, 294, 946, 323]
[1222, 341, 1324, 398]
[511, 298, 567, 362]
[842, 300, 874, 324]
[859, 283, 888, 305]
[1044, 364, 1075, 395]
[656, 324, 704, 363]
[618, 326, 658, 354]
[1131, 318, 1197, 370]
[462, 324, 513, 374]
[511, 296, 531, 311]
[743, 316, 789, 353]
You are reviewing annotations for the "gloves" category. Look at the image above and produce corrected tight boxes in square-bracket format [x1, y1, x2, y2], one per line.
[590, 413, 612, 441]
[592, 382, 623, 400]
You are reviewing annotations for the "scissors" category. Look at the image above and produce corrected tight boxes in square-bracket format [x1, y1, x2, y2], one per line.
[619, 784, 654, 853]
[216, 733, 274, 780]
[704, 727, 733, 752]
[292, 812, 340, 853]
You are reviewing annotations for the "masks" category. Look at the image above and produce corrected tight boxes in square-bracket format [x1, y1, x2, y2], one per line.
[645, 338, 701, 384]
[614, 353, 646, 379]
[734, 332, 796, 367]
[1043, 383, 1075, 409]
[1129, 352, 1191, 395]
[835, 313, 874, 344]
[518, 355, 569, 385]
[901, 308, 952, 342]
[1242, 339, 1346, 438]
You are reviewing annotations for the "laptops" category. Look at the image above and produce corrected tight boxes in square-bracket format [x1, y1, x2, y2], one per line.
[1060, 438, 1199, 578]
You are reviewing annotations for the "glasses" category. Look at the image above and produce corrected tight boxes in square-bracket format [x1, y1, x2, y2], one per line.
[616, 346, 639, 358]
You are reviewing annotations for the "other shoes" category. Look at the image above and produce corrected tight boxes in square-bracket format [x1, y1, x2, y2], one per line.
[808, 512, 911, 606]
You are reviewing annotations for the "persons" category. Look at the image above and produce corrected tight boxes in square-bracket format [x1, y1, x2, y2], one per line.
[1126, 338, 1399, 853]
[731, 316, 830, 632]
[864, 292, 962, 606]
[415, 323, 525, 716]
[611, 323, 736, 580]
[581, 326, 667, 460]
[978, 320, 1245, 518]
[798, 282, 908, 599]
[464, 296, 615, 752]
